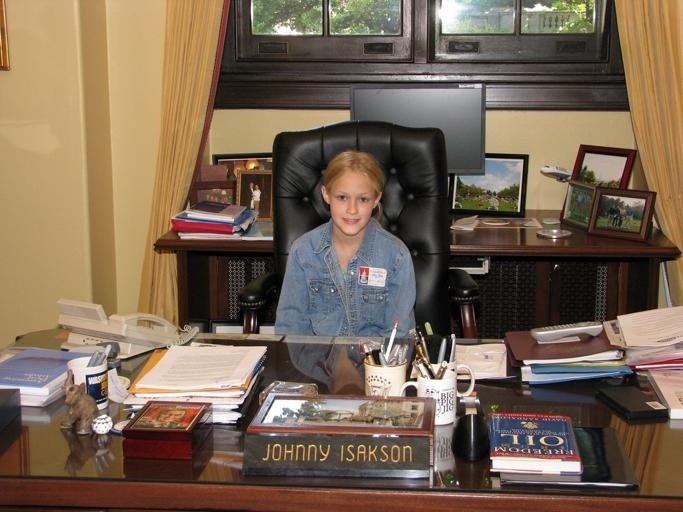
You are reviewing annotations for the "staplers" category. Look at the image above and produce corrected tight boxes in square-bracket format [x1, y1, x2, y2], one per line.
[96, 342, 122, 369]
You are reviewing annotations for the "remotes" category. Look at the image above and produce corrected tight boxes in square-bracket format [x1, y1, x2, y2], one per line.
[530, 320, 603, 341]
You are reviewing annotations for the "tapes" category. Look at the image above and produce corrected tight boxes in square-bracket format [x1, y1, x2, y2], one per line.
[465, 407, 477, 415]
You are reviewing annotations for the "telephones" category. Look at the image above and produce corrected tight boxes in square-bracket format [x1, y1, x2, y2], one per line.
[57, 298, 179, 360]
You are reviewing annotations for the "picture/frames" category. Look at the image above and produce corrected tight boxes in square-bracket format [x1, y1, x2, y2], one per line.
[238, 168, 273, 222]
[560, 182, 596, 230]
[212, 153, 273, 178]
[588, 187, 657, 242]
[446, 152, 530, 217]
[189, 179, 237, 209]
[571, 144, 637, 190]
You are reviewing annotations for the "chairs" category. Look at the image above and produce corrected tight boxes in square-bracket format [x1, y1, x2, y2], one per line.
[237, 120, 482, 339]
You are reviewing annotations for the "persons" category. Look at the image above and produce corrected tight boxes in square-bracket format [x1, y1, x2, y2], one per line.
[249, 182, 264, 215]
[604, 202, 636, 231]
[284, 342, 411, 399]
[274, 151, 419, 336]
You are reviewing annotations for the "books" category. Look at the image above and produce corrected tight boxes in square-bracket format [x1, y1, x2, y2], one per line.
[488, 406, 583, 471]
[138, 339, 269, 426]
[22, 408, 77, 423]
[647, 369, 683, 417]
[666, 419, 683, 431]
[499, 472, 582, 483]
[167, 199, 256, 242]
[1, 343, 104, 408]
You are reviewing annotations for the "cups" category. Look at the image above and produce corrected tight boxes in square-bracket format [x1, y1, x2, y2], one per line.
[363, 357, 407, 399]
[434, 423, 456, 473]
[68, 355, 110, 411]
[399, 373, 458, 425]
[418, 362, 477, 401]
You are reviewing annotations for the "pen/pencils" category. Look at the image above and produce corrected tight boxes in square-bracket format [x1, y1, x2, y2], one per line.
[363, 319, 456, 379]
[87, 344, 112, 367]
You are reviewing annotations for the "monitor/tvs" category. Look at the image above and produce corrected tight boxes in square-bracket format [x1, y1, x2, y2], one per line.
[348, 82, 487, 176]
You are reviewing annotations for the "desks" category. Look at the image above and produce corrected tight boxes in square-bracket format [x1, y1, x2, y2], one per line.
[1, 338, 683, 512]
[154, 209, 680, 332]
